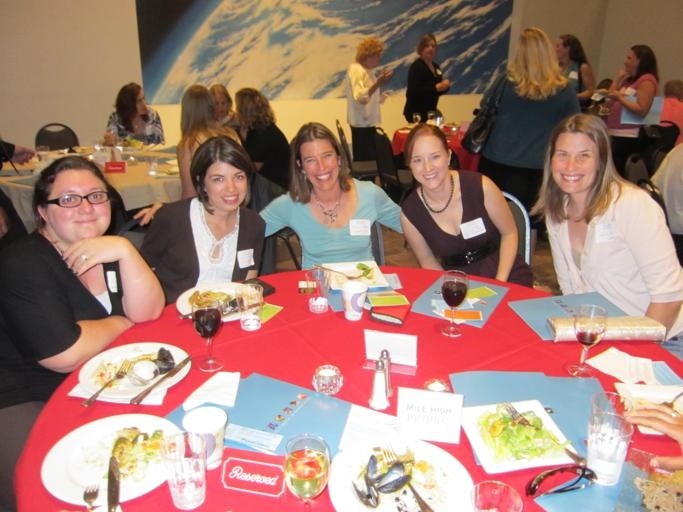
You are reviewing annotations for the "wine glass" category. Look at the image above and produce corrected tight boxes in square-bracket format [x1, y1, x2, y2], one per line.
[190, 298, 226, 372]
[404, 107, 473, 143]
[284, 435, 332, 511]
[437, 269, 468, 337]
[567, 303, 607, 382]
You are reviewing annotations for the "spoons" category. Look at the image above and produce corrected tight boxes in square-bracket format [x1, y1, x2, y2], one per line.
[659, 391, 683, 411]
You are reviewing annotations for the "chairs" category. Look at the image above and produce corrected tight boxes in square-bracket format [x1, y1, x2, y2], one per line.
[265, 118, 534, 272]
[33, 121, 82, 151]
[623, 119, 682, 227]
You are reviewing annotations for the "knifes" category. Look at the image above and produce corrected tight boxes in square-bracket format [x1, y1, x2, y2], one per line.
[127, 353, 191, 409]
[108, 455, 121, 512]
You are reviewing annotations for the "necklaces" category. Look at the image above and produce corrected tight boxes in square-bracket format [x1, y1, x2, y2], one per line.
[418, 174, 455, 215]
[311, 186, 346, 227]
[43, 225, 101, 295]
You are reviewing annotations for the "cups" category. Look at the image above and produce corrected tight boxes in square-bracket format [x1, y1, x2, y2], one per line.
[237, 285, 263, 331]
[162, 405, 229, 512]
[583, 390, 637, 488]
[304, 268, 367, 320]
[34, 146, 51, 167]
[475, 480, 523, 511]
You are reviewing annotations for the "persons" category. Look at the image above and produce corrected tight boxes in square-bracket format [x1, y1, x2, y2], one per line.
[138, 135, 268, 308]
[0, 139, 36, 245]
[101, 83, 166, 145]
[131, 121, 406, 271]
[208, 83, 240, 140]
[174, 85, 244, 202]
[528, 112, 683, 365]
[0, 155, 166, 511]
[231, 88, 290, 213]
[397, 122, 534, 287]
[620, 396, 682, 473]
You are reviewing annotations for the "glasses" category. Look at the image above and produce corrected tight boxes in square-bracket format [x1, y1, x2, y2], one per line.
[40, 190, 112, 208]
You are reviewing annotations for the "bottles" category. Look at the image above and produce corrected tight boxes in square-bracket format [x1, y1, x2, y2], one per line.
[368, 350, 394, 411]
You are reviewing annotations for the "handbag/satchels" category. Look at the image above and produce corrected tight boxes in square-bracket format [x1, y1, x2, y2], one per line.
[460, 69, 511, 155]
[575, 61, 607, 110]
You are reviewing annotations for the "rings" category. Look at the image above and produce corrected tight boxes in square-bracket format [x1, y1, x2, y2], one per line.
[670, 408, 679, 418]
[80, 254, 91, 265]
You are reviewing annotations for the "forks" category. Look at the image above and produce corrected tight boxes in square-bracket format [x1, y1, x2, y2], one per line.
[83, 358, 132, 408]
[84, 484, 99, 511]
[380, 446, 434, 512]
[505, 404, 589, 469]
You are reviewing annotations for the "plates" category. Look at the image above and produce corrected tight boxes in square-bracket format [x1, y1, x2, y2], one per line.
[612, 382, 683, 435]
[62, 146, 181, 177]
[320, 259, 392, 293]
[327, 439, 476, 511]
[79, 342, 192, 395]
[175, 284, 262, 322]
[38, 412, 185, 508]
[459, 400, 583, 474]
